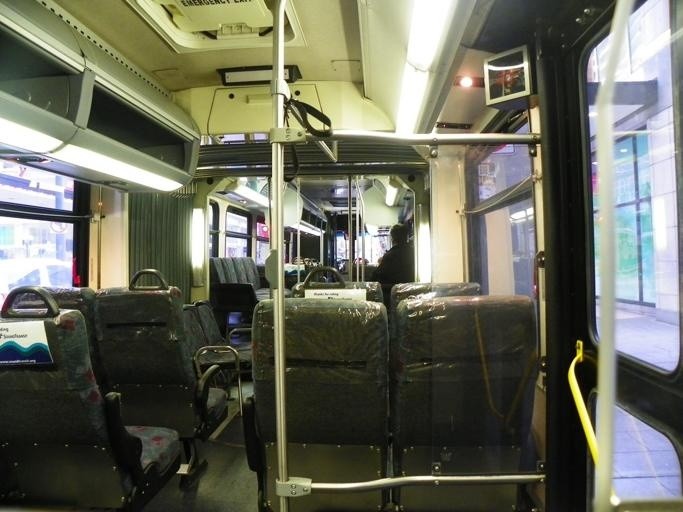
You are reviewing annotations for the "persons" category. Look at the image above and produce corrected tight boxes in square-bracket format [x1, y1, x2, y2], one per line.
[370, 224, 415, 300]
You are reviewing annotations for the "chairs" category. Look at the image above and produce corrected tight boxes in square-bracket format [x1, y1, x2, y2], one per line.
[246, 257, 545, 511]
[1, 257, 261, 511]
